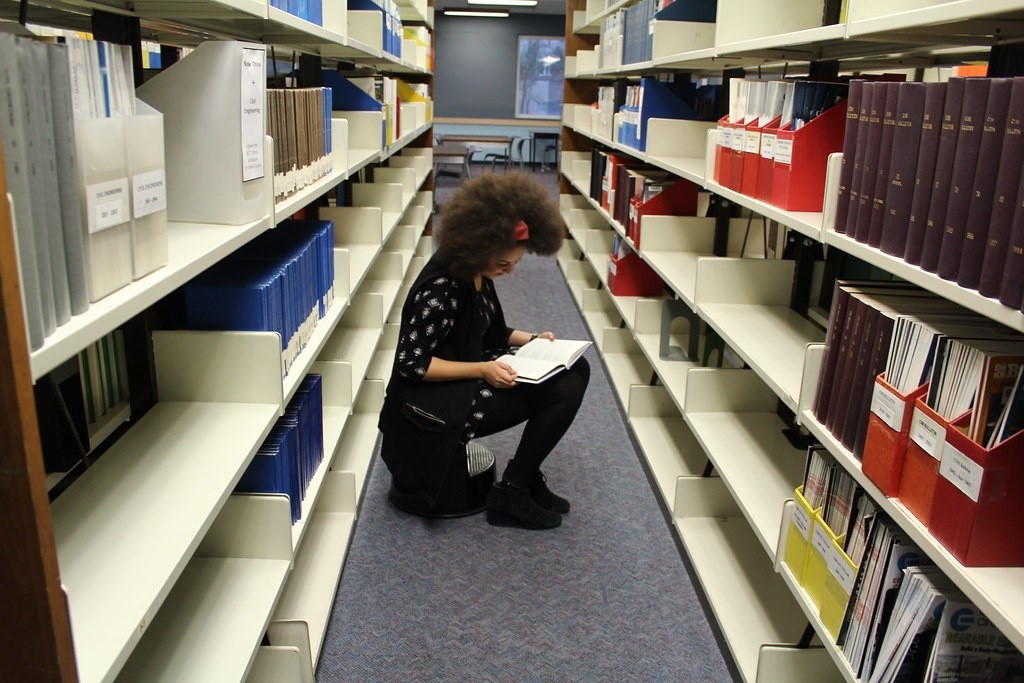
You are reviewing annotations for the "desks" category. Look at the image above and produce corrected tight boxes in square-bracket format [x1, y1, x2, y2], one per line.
[529, 129, 560, 173]
[433, 144, 472, 182]
[441, 133, 512, 176]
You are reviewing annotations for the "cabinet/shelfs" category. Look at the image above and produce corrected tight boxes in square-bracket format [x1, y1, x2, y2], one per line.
[0, 0, 437, 683]
[557, 0, 1024, 683]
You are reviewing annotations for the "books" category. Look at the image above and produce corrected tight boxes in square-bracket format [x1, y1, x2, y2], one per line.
[269, 0, 434, 72]
[336, 73, 434, 150]
[494, 336, 594, 385]
[0, 32, 336, 525]
[587, 0, 1023, 682]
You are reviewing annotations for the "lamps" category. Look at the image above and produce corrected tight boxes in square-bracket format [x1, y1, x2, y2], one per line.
[443, 0, 539, 18]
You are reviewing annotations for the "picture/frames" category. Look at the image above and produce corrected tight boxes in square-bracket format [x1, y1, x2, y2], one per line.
[514, 34, 565, 122]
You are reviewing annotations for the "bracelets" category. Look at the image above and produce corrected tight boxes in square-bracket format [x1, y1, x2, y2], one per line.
[530, 332, 539, 342]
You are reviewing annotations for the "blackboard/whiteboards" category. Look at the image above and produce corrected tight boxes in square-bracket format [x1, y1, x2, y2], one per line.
[432, 10, 566, 127]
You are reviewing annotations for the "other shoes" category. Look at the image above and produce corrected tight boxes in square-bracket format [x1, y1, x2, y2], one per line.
[502, 460, 571, 512]
[486, 482, 561, 529]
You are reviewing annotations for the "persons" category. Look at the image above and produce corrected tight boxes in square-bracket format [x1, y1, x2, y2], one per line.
[378, 172, 593, 532]
